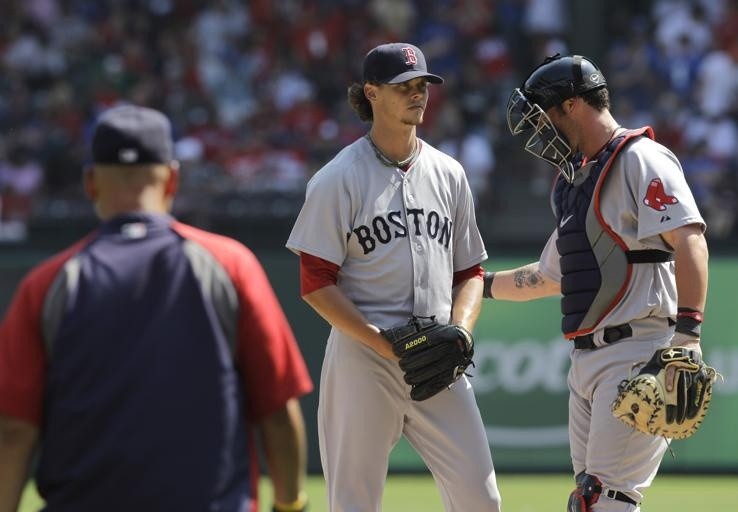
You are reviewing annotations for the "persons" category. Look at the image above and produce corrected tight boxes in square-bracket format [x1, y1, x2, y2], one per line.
[286, 42, 506, 510]
[1, 1, 738, 242]
[0, 112, 316, 511]
[482, 54, 709, 508]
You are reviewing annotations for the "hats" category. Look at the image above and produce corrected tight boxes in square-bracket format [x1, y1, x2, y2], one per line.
[92, 106, 172, 163]
[362, 43, 444, 85]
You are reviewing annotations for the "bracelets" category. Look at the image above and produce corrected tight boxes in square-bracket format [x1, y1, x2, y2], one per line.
[676, 310, 703, 323]
[675, 306, 704, 338]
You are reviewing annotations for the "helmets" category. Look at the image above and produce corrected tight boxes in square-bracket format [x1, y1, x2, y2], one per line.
[506, 53, 608, 184]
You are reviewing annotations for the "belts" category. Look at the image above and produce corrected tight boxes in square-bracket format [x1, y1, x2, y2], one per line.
[567, 317, 676, 349]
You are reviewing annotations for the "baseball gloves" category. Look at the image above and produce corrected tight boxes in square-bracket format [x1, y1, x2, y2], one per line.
[610, 346, 716, 439]
[379, 315, 474, 400]
